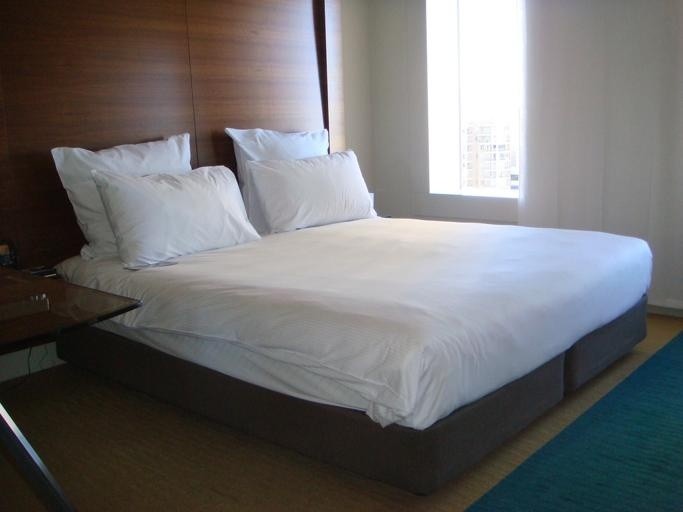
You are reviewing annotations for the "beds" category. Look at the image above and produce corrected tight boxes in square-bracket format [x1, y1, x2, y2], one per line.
[54, 213, 654, 497]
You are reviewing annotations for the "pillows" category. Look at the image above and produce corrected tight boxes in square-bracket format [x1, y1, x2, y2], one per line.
[90, 165, 261, 267]
[245, 149, 374, 236]
[225, 127, 330, 216]
[51, 133, 193, 264]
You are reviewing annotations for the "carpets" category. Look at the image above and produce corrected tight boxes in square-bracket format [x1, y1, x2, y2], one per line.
[460, 324, 681, 509]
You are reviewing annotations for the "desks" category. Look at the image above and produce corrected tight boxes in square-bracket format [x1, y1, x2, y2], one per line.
[0, 267, 141, 510]
